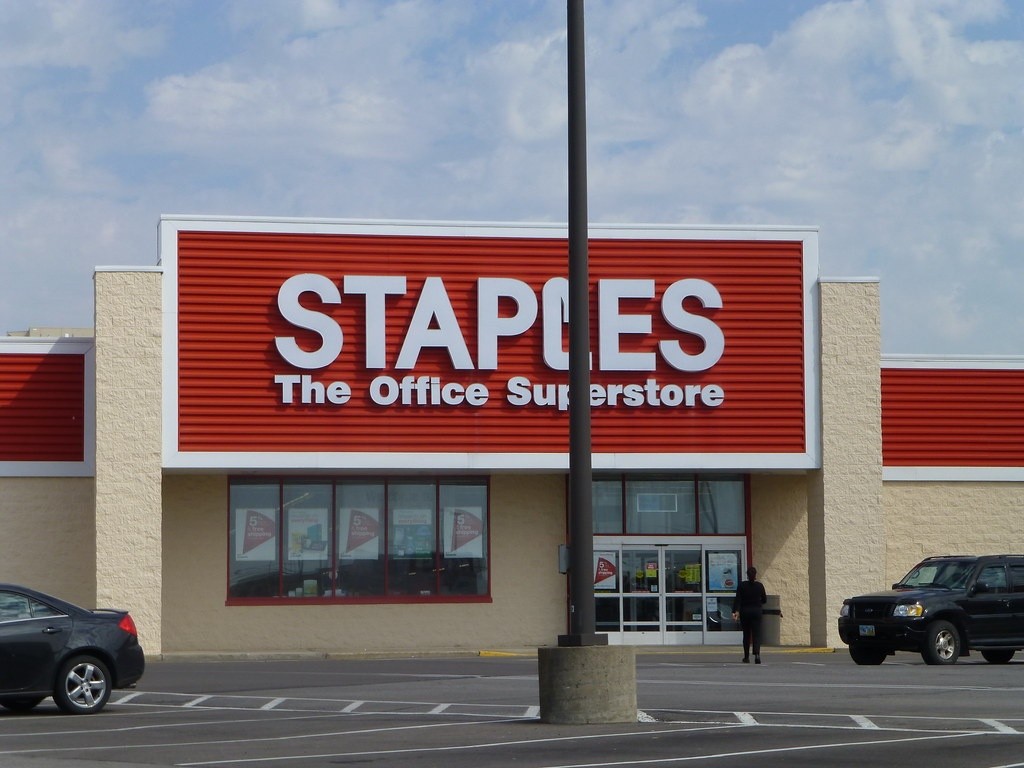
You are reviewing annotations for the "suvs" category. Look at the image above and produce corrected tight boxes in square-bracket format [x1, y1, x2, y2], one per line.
[837, 553, 1023, 666]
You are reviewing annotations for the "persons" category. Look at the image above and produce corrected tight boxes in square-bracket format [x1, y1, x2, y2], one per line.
[732, 567, 767, 664]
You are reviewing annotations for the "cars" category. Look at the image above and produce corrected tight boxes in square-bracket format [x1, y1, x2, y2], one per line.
[0, 584, 146, 718]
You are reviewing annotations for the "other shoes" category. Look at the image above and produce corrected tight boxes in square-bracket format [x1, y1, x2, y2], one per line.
[755, 654, 761, 664]
[743, 658, 750, 663]
